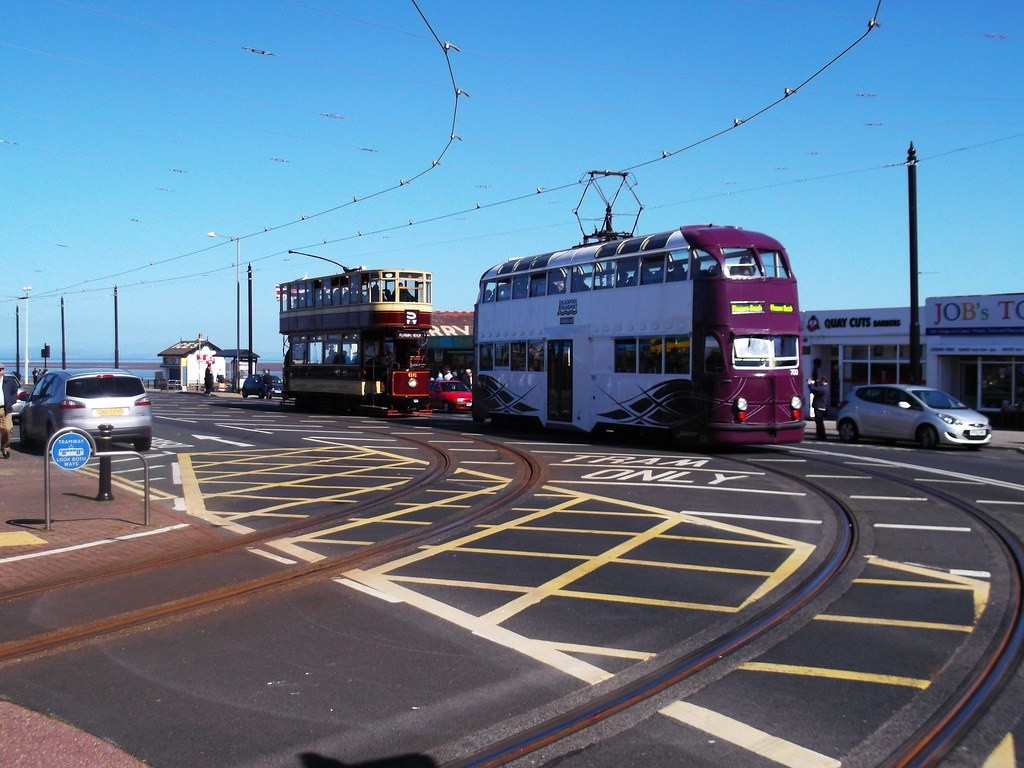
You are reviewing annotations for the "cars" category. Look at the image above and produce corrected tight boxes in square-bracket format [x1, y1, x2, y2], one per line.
[1, 371, 31, 425]
[835, 383, 990, 451]
[18, 367, 153, 453]
[242, 374, 284, 400]
[428, 380, 472, 415]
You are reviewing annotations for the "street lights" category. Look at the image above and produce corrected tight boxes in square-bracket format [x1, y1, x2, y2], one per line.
[22, 287, 32, 383]
[208, 233, 240, 392]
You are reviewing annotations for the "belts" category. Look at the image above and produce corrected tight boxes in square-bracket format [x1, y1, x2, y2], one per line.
[0, 405, 5, 408]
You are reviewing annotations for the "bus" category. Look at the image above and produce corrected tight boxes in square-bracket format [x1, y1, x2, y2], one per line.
[275, 248, 434, 418]
[470, 172, 806, 453]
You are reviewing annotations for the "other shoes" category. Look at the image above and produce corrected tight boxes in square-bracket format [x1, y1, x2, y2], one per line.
[3, 452, 10, 458]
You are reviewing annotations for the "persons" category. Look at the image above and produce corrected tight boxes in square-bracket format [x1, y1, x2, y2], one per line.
[32, 368, 48, 385]
[706, 351, 724, 373]
[294, 283, 417, 308]
[325, 349, 357, 363]
[807, 377, 830, 440]
[616, 348, 689, 374]
[263, 368, 273, 399]
[0, 363, 18, 458]
[618, 258, 756, 286]
[516, 354, 524, 370]
[436, 369, 473, 390]
[382, 346, 393, 391]
[203, 363, 211, 396]
[484, 276, 589, 302]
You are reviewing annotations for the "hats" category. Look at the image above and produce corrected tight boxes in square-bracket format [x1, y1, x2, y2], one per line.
[0, 364, 4, 368]
